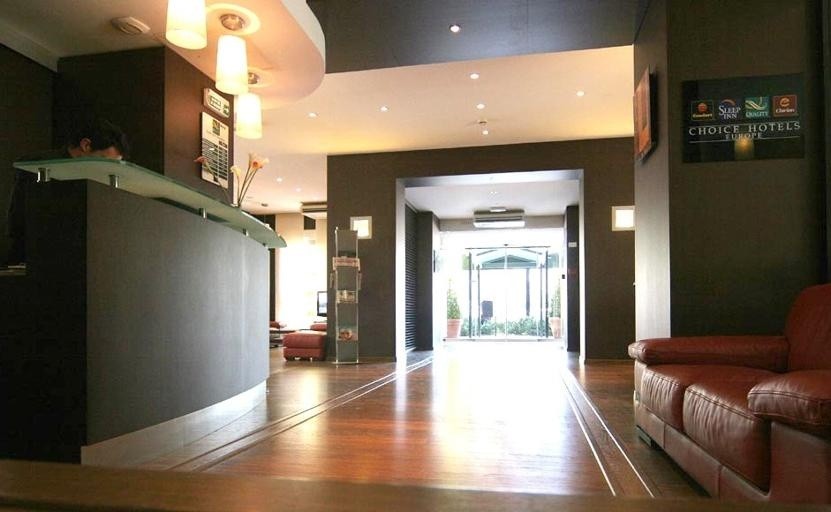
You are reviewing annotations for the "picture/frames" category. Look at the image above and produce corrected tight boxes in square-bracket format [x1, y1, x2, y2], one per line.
[632, 66, 658, 164]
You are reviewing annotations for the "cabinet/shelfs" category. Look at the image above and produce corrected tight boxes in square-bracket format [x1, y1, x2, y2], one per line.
[332, 226, 360, 365]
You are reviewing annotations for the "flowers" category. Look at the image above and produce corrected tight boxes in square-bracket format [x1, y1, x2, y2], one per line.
[194, 153, 269, 204]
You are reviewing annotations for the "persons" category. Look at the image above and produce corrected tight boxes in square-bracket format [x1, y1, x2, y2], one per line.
[3, 118, 130, 270]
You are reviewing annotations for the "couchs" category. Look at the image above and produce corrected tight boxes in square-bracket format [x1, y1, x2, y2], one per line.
[628, 284, 831, 503]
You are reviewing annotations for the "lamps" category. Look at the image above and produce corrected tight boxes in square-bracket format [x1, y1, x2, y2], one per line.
[233, 72, 263, 140]
[214, 14, 245, 95]
[165, 0, 207, 49]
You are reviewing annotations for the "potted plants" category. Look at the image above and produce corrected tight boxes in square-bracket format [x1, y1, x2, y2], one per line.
[448, 295, 463, 338]
[546, 288, 561, 338]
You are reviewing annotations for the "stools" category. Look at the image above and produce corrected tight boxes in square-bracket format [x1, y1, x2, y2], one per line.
[283, 322, 327, 362]
